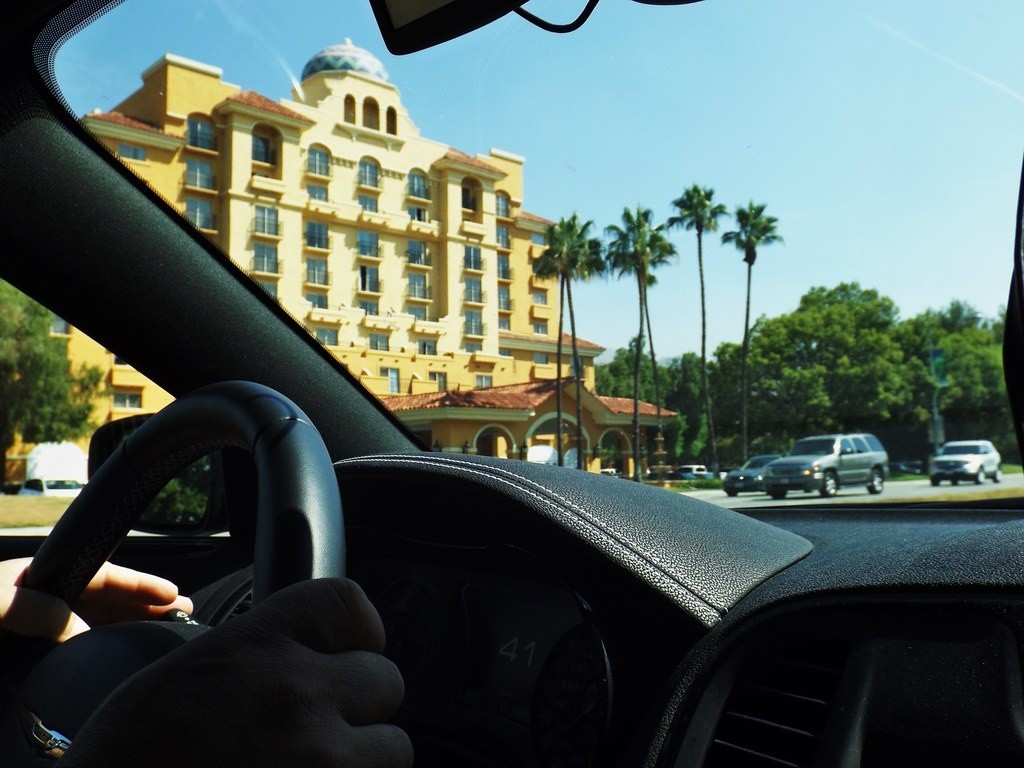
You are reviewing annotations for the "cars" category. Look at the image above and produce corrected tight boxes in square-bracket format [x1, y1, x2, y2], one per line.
[723, 455, 782, 497]
[17, 477, 83, 497]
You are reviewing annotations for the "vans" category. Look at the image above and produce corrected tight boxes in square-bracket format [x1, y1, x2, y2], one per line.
[676, 465, 707, 474]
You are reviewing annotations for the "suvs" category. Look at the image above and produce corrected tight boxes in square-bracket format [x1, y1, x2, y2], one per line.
[928, 440, 1002, 486]
[762, 433, 889, 500]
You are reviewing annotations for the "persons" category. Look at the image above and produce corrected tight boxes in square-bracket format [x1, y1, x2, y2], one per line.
[0, 555, 414, 768]
